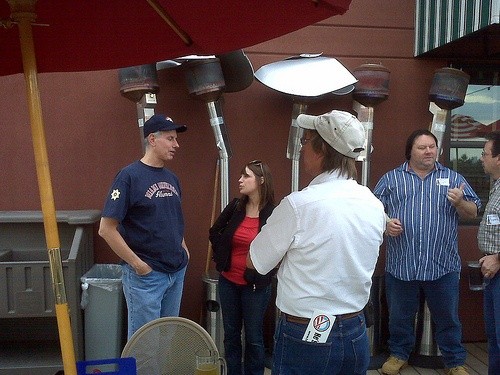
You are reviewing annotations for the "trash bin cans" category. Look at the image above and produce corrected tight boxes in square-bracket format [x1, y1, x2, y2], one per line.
[202, 275, 245, 375]
[80, 263, 124, 374]
[412, 294, 443, 368]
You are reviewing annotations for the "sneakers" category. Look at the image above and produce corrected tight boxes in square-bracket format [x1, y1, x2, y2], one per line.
[381, 354, 411, 375]
[444, 365, 470, 375]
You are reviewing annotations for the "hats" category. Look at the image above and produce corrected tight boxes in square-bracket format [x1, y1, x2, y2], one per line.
[143, 114, 188, 139]
[296, 110, 365, 158]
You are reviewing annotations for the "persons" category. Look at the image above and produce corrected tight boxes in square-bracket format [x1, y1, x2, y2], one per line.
[98, 113, 190, 375]
[372, 128, 481, 375]
[248, 109, 386, 375]
[210, 159, 277, 375]
[477, 132, 500, 375]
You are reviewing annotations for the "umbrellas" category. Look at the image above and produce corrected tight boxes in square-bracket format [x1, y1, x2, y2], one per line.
[0, 0, 352, 375]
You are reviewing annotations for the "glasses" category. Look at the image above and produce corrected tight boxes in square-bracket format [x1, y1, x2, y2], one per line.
[299, 137, 316, 145]
[249, 159, 264, 178]
[481, 151, 498, 158]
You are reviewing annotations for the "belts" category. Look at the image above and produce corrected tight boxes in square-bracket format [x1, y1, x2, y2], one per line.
[286, 309, 363, 325]
